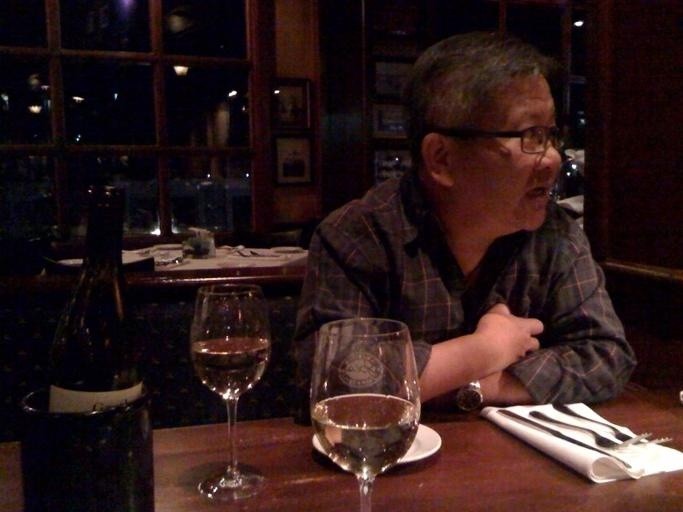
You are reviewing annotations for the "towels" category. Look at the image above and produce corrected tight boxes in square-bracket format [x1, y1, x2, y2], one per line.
[480, 402, 683, 485]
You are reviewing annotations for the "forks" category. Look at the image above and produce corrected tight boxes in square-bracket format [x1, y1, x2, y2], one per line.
[530, 400, 675, 447]
[237, 250, 280, 258]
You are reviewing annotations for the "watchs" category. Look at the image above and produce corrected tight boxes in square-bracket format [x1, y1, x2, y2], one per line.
[456, 380, 485, 410]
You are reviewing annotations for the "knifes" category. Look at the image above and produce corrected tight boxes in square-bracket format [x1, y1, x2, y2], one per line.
[496, 408, 632, 473]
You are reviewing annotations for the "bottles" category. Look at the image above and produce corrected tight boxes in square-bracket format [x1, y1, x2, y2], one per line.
[42, 184, 144, 410]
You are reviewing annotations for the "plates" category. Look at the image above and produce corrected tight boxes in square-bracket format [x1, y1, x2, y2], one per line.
[312, 422, 443, 468]
[59, 258, 83, 266]
[273, 246, 303, 253]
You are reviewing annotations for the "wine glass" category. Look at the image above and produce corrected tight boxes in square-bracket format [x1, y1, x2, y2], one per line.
[308, 317, 423, 512]
[188, 283, 273, 501]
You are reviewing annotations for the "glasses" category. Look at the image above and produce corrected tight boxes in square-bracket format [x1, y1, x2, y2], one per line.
[429, 125, 567, 154]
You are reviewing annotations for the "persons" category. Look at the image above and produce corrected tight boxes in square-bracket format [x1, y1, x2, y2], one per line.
[287, 26, 640, 428]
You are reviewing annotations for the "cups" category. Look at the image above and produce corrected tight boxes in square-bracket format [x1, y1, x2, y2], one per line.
[17, 390, 157, 512]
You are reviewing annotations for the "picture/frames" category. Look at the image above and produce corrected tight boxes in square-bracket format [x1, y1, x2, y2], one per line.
[371, 99, 413, 144]
[371, 55, 415, 99]
[272, 134, 314, 186]
[371, 144, 414, 184]
[270, 77, 311, 131]
